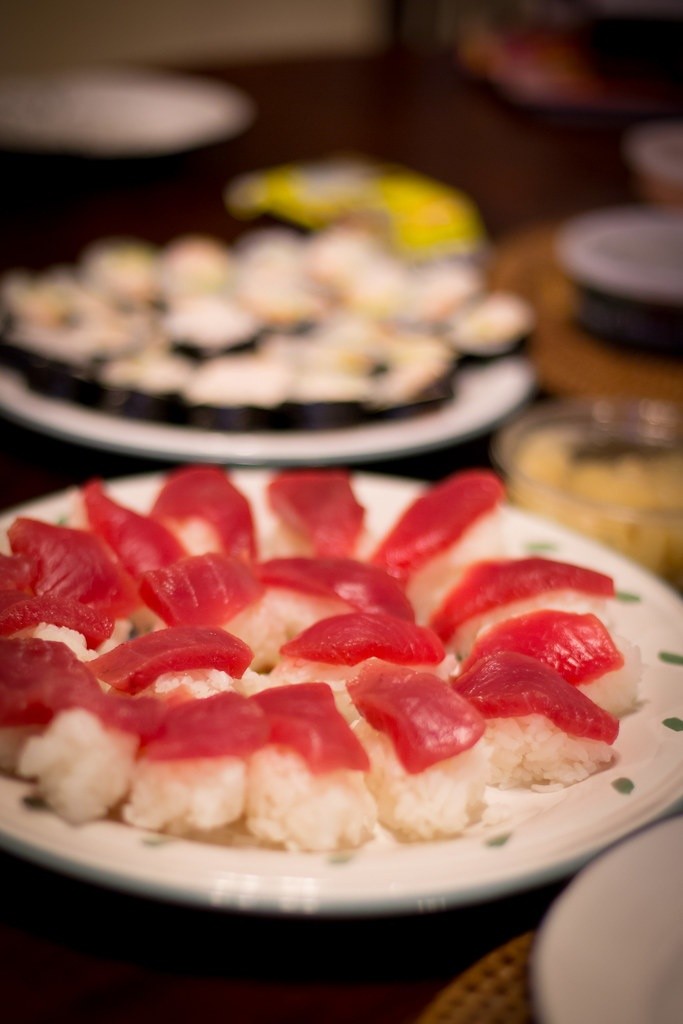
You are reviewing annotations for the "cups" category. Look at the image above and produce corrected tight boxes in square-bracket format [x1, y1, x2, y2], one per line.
[490, 398, 683, 596]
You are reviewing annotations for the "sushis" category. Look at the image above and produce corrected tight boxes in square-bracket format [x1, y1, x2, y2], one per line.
[0, 466, 647, 855]
[0, 230, 534, 433]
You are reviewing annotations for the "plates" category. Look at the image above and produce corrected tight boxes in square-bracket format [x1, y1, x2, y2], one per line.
[0, 244, 536, 468]
[530, 817, 683, 1024]
[0, 467, 683, 918]
